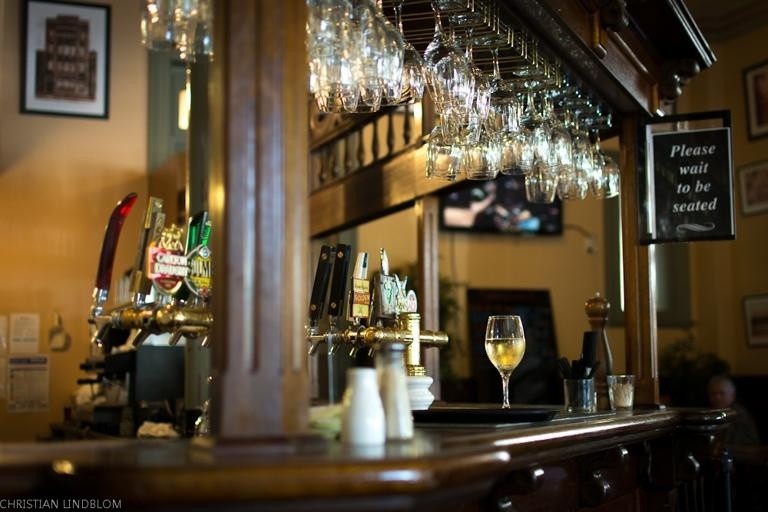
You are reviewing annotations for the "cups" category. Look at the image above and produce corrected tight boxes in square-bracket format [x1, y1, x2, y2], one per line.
[607, 374, 635, 412]
[306, 1, 383, 113]
[564, 378, 598, 413]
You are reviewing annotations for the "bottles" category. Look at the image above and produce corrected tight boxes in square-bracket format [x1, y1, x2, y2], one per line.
[381, 343, 414, 441]
[339, 368, 387, 447]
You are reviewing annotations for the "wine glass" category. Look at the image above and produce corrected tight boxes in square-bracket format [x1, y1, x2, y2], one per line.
[385, 0, 423, 104]
[484, 314, 526, 410]
[424, 1, 621, 207]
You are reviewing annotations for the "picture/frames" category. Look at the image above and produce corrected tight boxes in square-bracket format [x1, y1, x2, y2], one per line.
[19, 0, 112, 119]
[742, 294, 768, 349]
[736, 57, 766, 216]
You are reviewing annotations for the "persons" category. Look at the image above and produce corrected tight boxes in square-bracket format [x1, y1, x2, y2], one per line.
[707, 374, 759, 447]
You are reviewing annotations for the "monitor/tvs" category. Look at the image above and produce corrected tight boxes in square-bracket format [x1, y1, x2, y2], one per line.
[437, 172, 564, 237]
